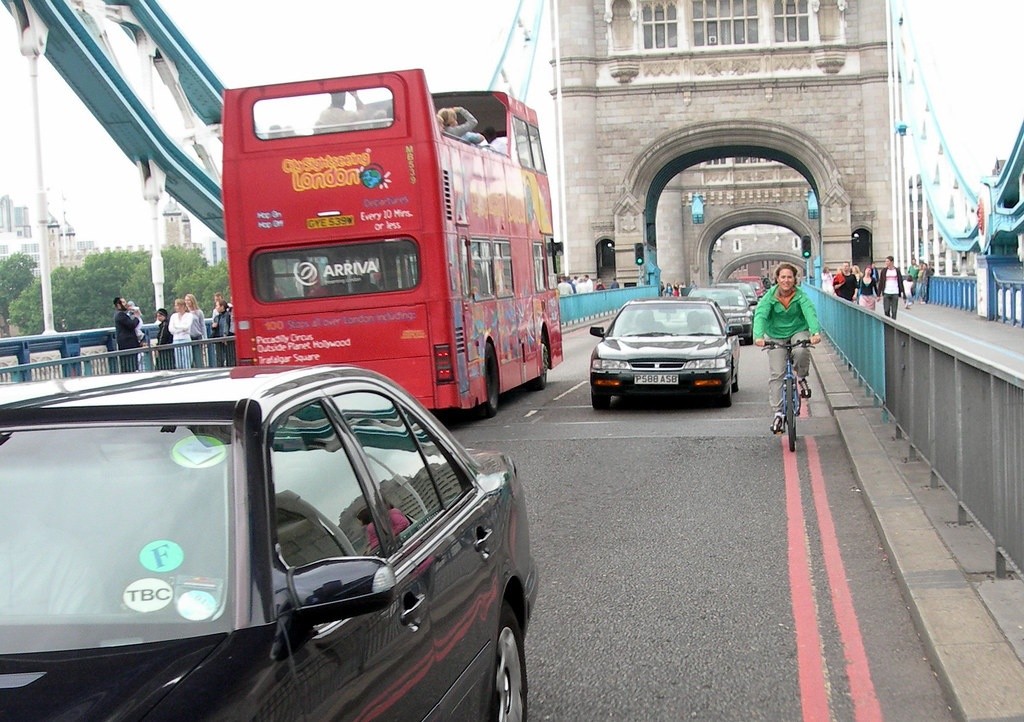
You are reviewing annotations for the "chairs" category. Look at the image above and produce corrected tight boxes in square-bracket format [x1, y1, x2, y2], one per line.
[268, 125, 282, 138]
[283, 126, 296, 137]
[315, 108, 354, 133]
[724, 296, 739, 305]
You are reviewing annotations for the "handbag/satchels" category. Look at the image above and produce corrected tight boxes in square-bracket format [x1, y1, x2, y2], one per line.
[210, 315, 220, 338]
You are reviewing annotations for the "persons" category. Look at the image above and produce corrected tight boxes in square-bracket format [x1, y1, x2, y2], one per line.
[877, 256, 906, 320]
[268, 126, 283, 138]
[858, 267, 879, 310]
[833, 262, 863, 302]
[358, 498, 410, 554]
[155, 294, 208, 370]
[908, 259, 931, 304]
[315, 90, 393, 132]
[308, 272, 378, 296]
[753, 264, 822, 435]
[870, 264, 879, 284]
[661, 281, 686, 297]
[594, 279, 606, 291]
[902, 275, 914, 309]
[821, 267, 833, 293]
[211, 294, 236, 367]
[558, 275, 593, 295]
[113, 296, 147, 373]
[691, 281, 697, 287]
[611, 278, 619, 289]
[437, 107, 484, 146]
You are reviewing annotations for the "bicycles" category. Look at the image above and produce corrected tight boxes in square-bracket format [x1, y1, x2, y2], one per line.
[756, 338, 817, 453]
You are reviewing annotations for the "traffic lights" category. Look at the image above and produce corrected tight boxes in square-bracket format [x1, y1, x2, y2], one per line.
[801, 235, 811, 258]
[636, 243, 645, 265]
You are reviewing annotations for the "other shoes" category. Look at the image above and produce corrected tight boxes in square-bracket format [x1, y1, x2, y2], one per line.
[772, 416, 783, 433]
[920, 301, 927, 305]
[798, 378, 811, 398]
[140, 334, 147, 343]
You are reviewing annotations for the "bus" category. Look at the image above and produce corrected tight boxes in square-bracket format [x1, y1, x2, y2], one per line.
[218, 68, 566, 426]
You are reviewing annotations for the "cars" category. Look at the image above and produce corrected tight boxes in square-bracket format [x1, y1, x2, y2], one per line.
[1, 363, 541, 722]
[686, 286, 757, 346]
[588, 297, 744, 410]
[711, 276, 766, 315]
